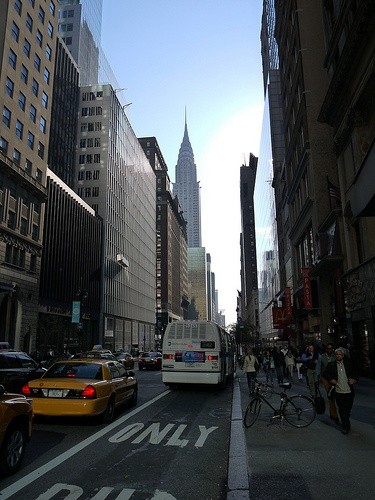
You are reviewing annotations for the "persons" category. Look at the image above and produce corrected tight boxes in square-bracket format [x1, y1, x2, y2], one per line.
[326, 348, 360, 434]
[243, 343, 308, 395]
[300, 342, 352, 420]
[48, 348, 54, 361]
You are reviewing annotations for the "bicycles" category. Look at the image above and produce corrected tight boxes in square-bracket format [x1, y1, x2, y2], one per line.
[242, 377, 317, 429]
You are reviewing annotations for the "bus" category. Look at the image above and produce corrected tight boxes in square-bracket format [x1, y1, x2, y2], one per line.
[161, 320, 235, 390]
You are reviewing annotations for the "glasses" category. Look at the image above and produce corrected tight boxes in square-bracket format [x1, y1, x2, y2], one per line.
[327, 348, 332, 349]
[336, 354, 343, 355]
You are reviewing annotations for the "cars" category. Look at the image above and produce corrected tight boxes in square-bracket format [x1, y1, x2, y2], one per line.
[0, 384, 35, 475]
[137, 352, 163, 372]
[80, 344, 118, 362]
[22, 360, 138, 427]
[112, 352, 134, 370]
[0, 342, 48, 393]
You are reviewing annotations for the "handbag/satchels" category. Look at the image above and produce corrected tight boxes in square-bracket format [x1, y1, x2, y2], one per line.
[300, 366, 305, 373]
[327, 385, 335, 400]
[315, 387, 326, 415]
[254, 357, 260, 372]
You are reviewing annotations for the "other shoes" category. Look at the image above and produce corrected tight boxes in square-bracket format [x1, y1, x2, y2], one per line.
[348, 420, 351, 430]
[250, 392, 255, 397]
[342, 429, 349, 434]
[330, 415, 335, 420]
